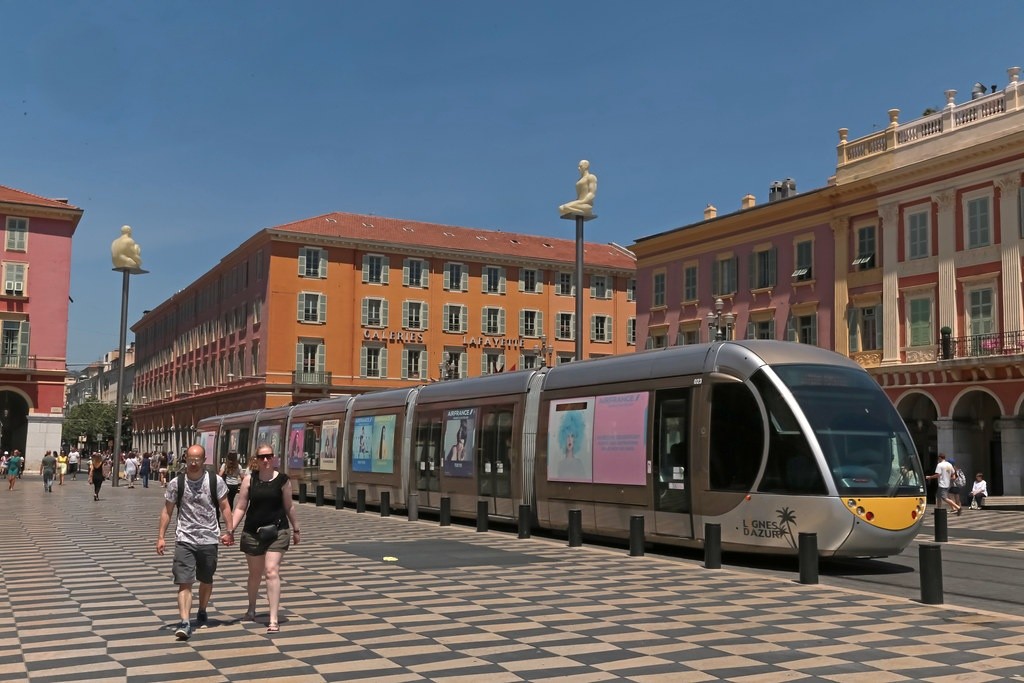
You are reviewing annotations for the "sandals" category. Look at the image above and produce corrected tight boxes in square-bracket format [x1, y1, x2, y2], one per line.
[232, 613, 256, 623]
[266, 621, 280, 634]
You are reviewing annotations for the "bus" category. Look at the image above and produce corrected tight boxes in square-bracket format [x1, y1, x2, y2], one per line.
[198, 337, 928, 560]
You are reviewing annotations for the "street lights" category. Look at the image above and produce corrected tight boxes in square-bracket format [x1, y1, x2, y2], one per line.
[437, 351, 455, 379]
[532, 336, 553, 367]
[706, 299, 734, 341]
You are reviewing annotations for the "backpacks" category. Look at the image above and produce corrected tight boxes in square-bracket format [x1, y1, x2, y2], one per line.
[952, 469, 967, 487]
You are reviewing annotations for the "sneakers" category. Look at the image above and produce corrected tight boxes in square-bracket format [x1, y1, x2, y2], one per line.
[197, 611, 208, 629]
[175, 621, 192, 638]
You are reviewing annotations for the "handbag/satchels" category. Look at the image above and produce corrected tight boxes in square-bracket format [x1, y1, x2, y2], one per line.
[256, 524, 278, 543]
[222, 463, 227, 483]
[971, 496, 978, 510]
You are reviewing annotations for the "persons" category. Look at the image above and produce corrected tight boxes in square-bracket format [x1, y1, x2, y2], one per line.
[218, 449, 244, 518]
[557, 159, 597, 215]
[947, 460, 962, 514]
[220, 443, 301, 634]
[556, 410, 586, 481]
[110, 225, 142, 268]
[900, 466, 919, 486]
[89, 454, 107, 501]
[446, 420, 467, 461]
[0, 447, 187, 501]
[40, 450, 56, 492]
[258, 425, 388, 459]
[156, 444, 235, 638]
[7, 449, 22, 491]
[966, 472, 988, 510]
[926, 453, 963, 516]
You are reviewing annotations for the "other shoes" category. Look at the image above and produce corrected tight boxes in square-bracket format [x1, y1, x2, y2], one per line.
[978, 507, 982, 509]
[49, 485, 51, 492]
[969, 507, 971, 510]
[94, 494, 99, 501]
[143, 485, 149, 488]
[128, 485, 134, 489]
[948, 505, 963, 517]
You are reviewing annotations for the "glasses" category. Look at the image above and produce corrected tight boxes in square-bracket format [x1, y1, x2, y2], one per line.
[186, 457, 203, 463]
[257, 453, 274, 461]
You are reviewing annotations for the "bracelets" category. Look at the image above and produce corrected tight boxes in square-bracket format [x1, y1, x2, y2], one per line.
[294, 528, 301, 533]
[225, 529, 235, 535]
